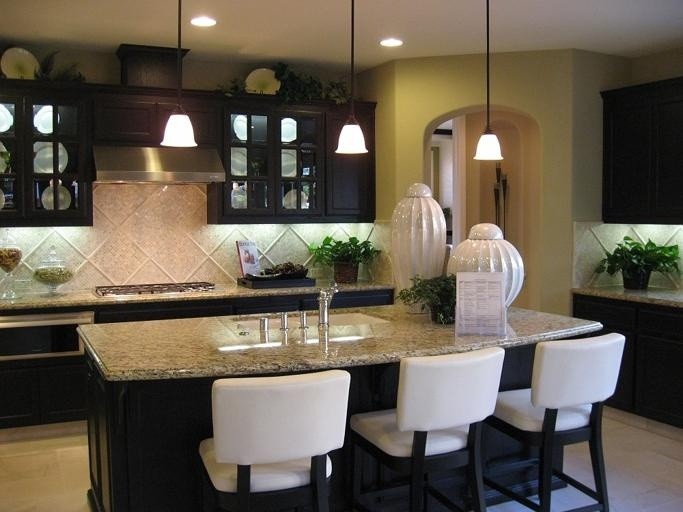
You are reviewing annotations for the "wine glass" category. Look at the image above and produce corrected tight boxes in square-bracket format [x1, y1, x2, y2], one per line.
[250, 157, 265, 175]
[30, 244, 77, 298]
[0, 227, 25, 301]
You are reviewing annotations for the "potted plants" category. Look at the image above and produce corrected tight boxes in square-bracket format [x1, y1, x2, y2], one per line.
[590, 233, 682, 293]
[395, 271, 459, 326]
[304, 232, 381, 284]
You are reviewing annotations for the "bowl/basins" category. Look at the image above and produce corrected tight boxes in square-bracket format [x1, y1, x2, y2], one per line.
[245, 271, 307, 280]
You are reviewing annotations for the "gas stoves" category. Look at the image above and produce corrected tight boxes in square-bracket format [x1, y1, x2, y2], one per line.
[93, 281, 215, 299]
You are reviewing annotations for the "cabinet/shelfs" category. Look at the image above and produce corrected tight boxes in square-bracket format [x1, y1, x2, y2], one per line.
[601, 76, 681, 226]
[206, 88, 323, 227]
[322, 93, 379, 224]
[572, 291, 639, 415]
[86, 80, 224, 150]
[0, 356, 89, 432]
[0, 79, 93, 226]
[635, 298, 682, 432]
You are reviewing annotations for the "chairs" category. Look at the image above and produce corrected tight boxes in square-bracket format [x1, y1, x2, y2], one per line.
[473, 331, 628, 512]
[342, 346, 504, 512]
[191, 364, 352, 511]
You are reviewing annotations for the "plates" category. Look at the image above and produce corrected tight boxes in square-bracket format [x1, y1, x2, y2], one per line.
[246, 67, 280, 96]
[33, 141, 69, 173]
[42, 185, 71, 210]
[281, 118, 299, 143]
[282, 189, 308, 209]
[33, 104, 62, 134]
[0, 189, 5, 209]
[231, 147, 248, 176]
[281, 149, 296, 178]
[0, 104, 14, 132]
[0, 142, 11, 173]
[234, 116, 248, 142]
[1, 47, 40, 80]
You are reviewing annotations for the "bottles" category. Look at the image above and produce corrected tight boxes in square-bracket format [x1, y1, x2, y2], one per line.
[231, 181, 247, 208]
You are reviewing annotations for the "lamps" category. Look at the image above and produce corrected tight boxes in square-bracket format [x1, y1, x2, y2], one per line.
[330, 0, 369, 157]
[471, 2, 506, 163]
[158, 0, 196, 147]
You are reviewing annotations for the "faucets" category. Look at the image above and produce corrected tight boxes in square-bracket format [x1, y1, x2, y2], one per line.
[315, 281, 340, 334]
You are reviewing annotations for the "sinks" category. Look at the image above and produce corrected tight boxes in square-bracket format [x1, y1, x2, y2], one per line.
[230, 307, 395, 332]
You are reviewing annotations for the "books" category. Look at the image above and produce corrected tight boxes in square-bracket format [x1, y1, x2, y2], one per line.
[235, 240, 261, 278]
